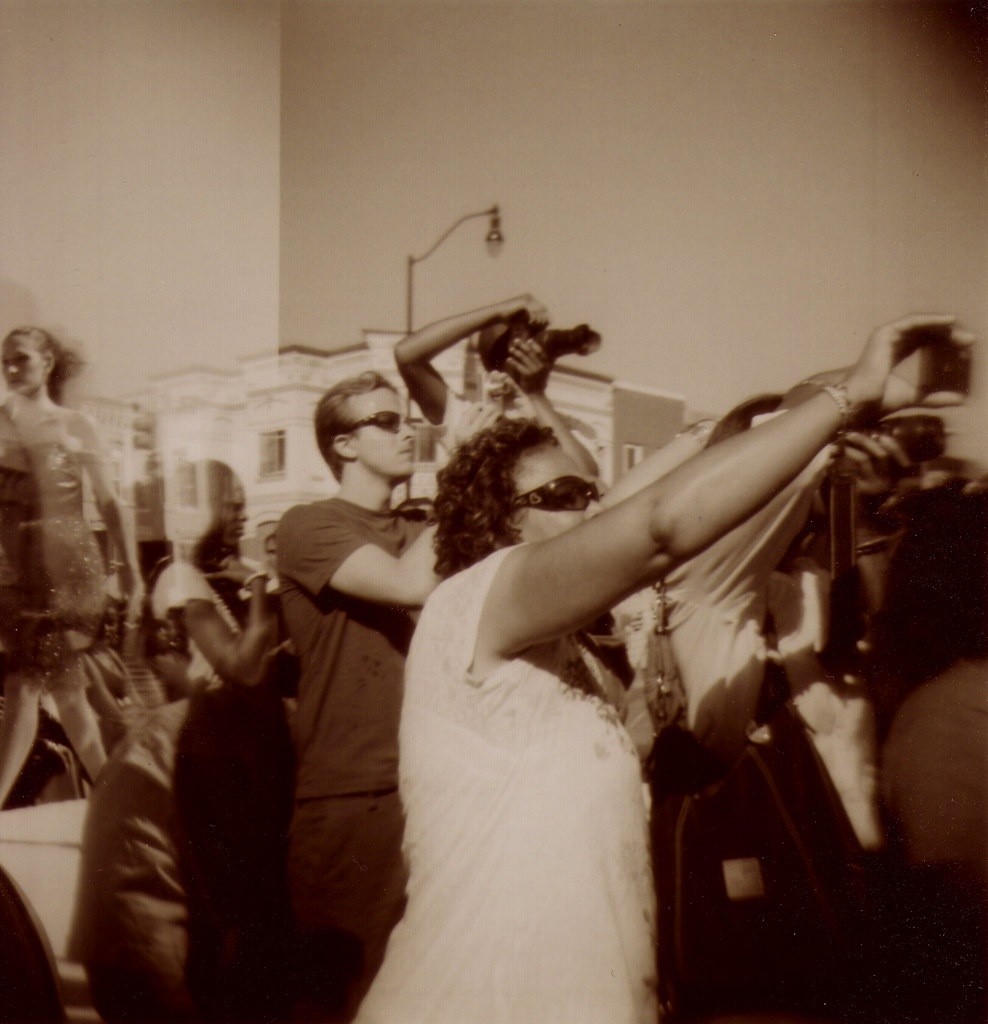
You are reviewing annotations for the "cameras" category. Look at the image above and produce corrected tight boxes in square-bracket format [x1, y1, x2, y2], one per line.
[860, 414, 947, 462]
[510, 315, 600, 359]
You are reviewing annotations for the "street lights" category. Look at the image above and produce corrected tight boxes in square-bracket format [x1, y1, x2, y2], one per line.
[404, 205, 504, 504]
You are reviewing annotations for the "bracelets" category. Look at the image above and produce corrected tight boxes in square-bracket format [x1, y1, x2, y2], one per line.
[822, 383, 851, 427]
[242, 570, 270, 592]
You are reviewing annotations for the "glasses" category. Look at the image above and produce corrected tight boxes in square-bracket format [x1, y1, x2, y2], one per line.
[508, 475, 599, 511]
[343, 410, 414, 434]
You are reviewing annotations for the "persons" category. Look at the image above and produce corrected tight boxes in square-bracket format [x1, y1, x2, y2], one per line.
[0, 460, 284, 1024]
[392, 290, 603, 485]
[392, 392, 987, 1024]
[348, 309, 980, 1024]
[0, 321, 148, 815]
[274, 367, 445, 1024]
